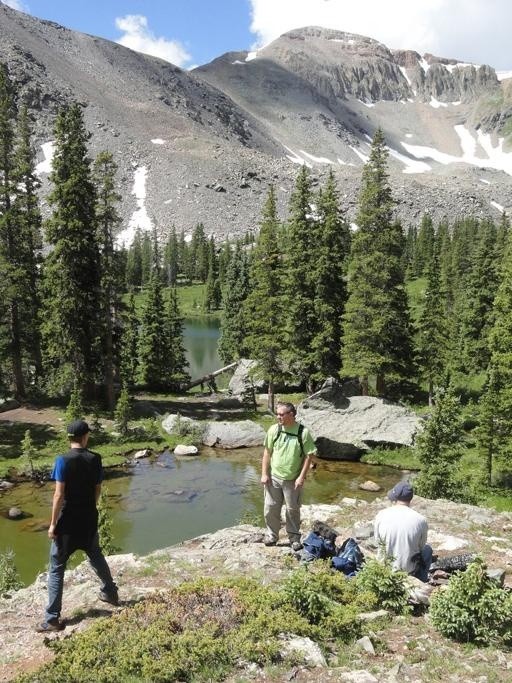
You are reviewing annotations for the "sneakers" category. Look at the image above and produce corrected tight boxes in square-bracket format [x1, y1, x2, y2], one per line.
[96, 590, 118, 605]
[34, 620, 59, 632]
[265, 539, 276, 546]
[290, 542, 303, 550]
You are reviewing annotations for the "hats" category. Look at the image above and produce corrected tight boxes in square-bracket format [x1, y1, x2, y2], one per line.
[67, 420, 91, 437]
[387, 482, 413, 500]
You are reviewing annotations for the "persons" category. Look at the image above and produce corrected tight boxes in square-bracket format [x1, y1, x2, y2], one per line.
[372, 480, 433, 581]
[260, 401, 318, 549]
[34, 420, 123, 632]
